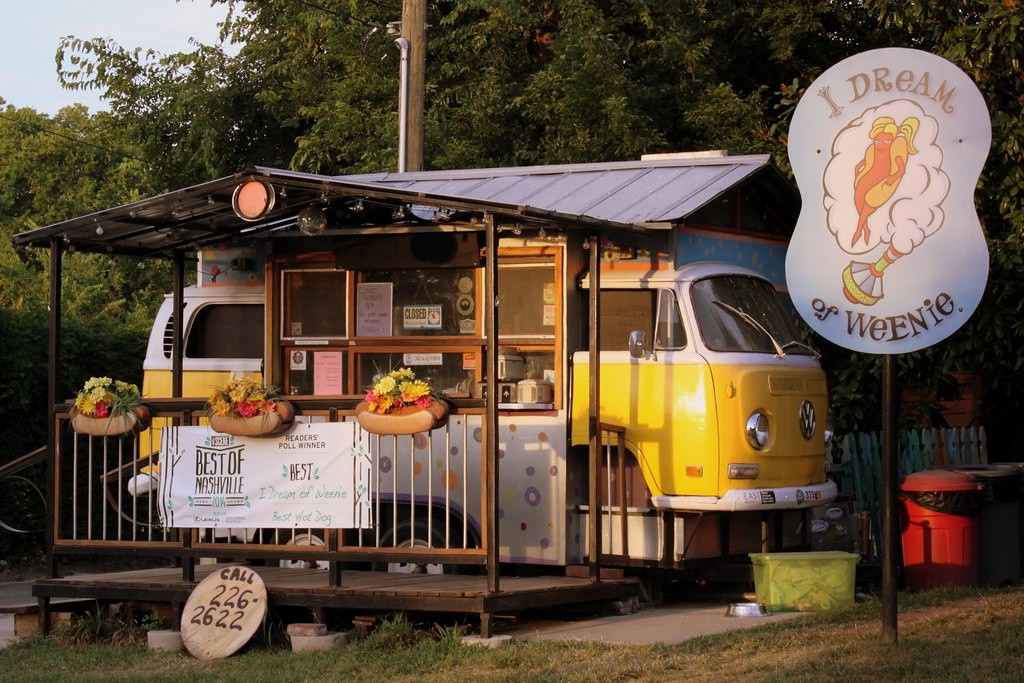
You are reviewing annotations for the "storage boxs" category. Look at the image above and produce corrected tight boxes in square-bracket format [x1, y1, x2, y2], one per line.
[747, 551, 861, 614]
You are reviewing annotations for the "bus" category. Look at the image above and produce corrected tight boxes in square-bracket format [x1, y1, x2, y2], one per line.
[140, 239, 843, 633]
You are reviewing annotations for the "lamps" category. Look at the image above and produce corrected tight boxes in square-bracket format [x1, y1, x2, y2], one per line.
[232, 176, 276, 221]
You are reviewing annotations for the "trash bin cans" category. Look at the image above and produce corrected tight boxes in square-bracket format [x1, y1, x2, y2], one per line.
[954, 461, 1024, 588]
[896, 467, 984, 593]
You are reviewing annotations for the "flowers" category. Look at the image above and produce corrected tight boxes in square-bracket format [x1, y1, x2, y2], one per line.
[361, 350, 455, 417]
[202, 369, 290, 437]
[64, 377, 159, 440]
